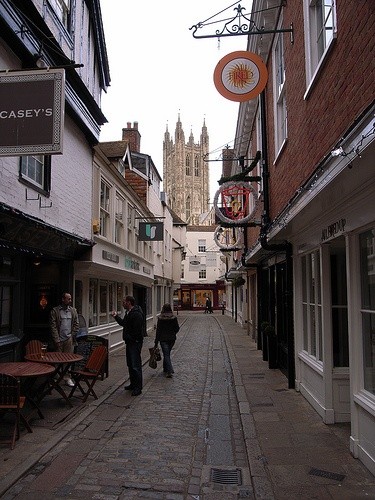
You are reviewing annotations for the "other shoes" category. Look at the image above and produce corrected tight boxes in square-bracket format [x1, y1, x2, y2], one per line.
[125, 384, 134, 390]
[166, 373, 173, 378]
[65, 379, 74, 386]
[131, 390, 142, 396]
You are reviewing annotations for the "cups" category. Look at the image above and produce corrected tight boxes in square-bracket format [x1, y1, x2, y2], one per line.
[40, 343, 48, 357]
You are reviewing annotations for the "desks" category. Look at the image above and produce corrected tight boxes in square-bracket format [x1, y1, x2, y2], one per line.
[0, 361, 55, 433]
[24, 352, 84, 408]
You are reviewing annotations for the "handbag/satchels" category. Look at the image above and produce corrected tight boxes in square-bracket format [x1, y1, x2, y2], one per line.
[148, 341, 162, 369]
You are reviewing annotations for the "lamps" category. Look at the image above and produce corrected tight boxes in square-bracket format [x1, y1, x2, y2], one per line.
[190, 255, 200, 265]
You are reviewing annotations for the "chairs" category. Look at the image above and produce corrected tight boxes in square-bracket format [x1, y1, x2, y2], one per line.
[0, 372, 26, 450]
[25, 340, 109, 403]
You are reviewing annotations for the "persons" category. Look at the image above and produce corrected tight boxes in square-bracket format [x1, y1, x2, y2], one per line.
[154, 303, 179, 379]
[203, 296, 213, 314]
[111, 296, 145, 397]
[50, 291, 80, 387]
